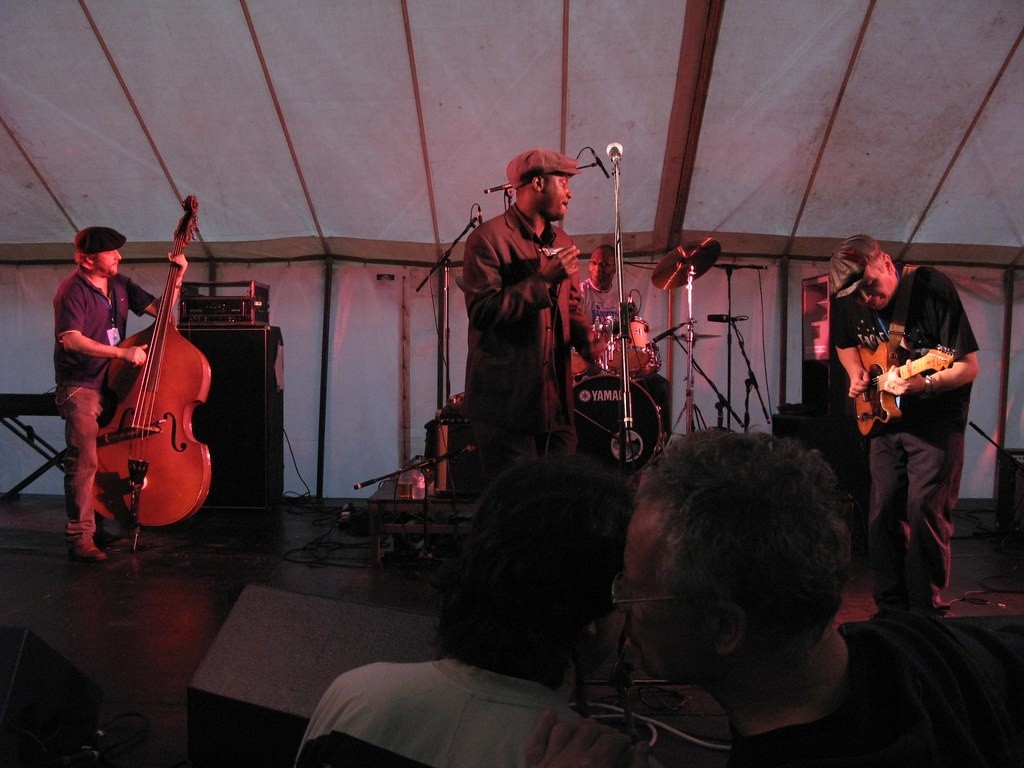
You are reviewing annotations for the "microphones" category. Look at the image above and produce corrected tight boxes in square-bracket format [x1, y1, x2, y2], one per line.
[707, 314, 742, 322]
[653, 324, 683, 343]
[463, 444, 476, 453]
[606, 143, 623, 165]
[628, 296, 632, 311]
[477, 206, 482, 225]
[591, 149, 610, 178]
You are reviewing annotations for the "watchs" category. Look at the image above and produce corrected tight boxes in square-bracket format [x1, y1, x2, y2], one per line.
[176, 281, 182, 289]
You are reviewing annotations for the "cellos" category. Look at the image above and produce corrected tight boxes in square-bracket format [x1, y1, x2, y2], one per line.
[92, 196, 211, 550]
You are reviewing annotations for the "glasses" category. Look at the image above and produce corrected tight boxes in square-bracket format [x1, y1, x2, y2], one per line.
[611, 571, 727, 615]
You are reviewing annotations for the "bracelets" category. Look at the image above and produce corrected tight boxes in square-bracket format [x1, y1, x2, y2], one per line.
[924, 375, 932, 392]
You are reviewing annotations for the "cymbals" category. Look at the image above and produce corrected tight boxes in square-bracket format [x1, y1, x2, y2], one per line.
[651, 237, 721, 290]
[663, 332, 723, 342]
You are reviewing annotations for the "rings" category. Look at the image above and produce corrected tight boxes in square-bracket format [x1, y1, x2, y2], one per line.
[891, 385, 895, 389]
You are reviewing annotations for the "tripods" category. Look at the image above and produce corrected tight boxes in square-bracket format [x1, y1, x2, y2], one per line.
[576, 162, 686, 744]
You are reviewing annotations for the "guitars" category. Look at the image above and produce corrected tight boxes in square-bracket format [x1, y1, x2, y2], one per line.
[855, 342, 956, 436]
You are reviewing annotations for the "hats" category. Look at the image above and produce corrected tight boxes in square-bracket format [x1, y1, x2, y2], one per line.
[74, 226, 127, 254]
[506, 150, 582, 189]
[829, 234, 878, 300]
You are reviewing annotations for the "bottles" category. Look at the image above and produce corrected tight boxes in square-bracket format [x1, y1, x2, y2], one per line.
[411, 475, 426, 499]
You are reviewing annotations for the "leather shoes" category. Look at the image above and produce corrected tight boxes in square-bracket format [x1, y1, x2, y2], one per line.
[69, 542, 107, 561]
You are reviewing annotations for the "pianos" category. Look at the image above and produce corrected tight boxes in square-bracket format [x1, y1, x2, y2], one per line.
[0, 393, 68, 501]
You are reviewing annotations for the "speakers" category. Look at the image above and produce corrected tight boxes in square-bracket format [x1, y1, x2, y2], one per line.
[186, 583, 441, 768]
[0, 626, 101, 768]
[801, 273, 856, 417]
[176, 324, 284, 512]
[425, 423, 477, 491]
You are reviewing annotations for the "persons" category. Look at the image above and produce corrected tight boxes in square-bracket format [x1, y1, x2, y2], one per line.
[579, 245, 617, 324]
[294, 457, 661, 768]
[54, 227, 188, 562]
[827, 235, 980, 616]
[525, 428, 1024, 768]
[462, 149, 605, 491]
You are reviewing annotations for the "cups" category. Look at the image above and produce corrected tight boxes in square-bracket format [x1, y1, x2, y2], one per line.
[543, 247, 563, 260]
[398, 482, 411, 498]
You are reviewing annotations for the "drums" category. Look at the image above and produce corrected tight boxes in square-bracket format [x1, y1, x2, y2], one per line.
[593, 315, 651, 372]
[572, 349, 602, 376]
[573, 373, 662, 476]
[638, 342, 662, 373]
